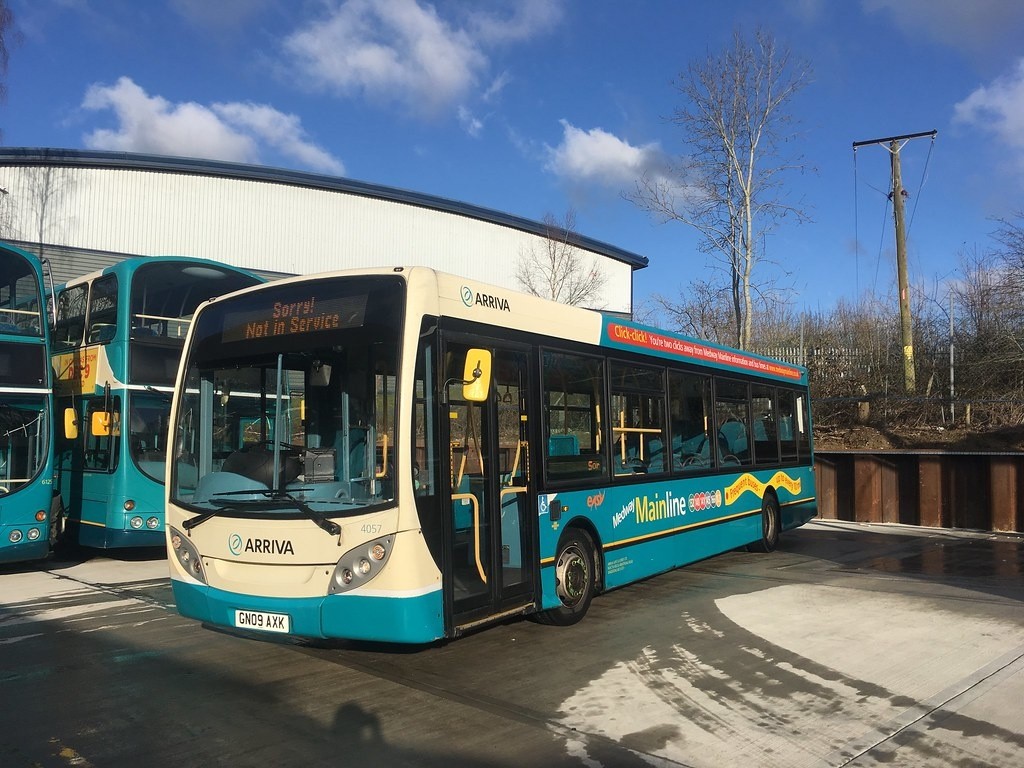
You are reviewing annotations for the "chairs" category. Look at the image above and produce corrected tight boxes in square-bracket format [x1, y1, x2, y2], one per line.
[671, 431, 683, 457]
[628, 457, 648, 475]
[615, 433, 638, 460]
[661, 458, 682, 474]
[702, 437, 724, 462]
[646, 435, 663, 456]
[721, 456, 743, 469]
[719, 416, 745, 453]
[133, 326, 159, 339]
[630, 419, 654, 441]
[683, 457, 706, 472]
[649, 458, 664, 474]
[98, 325, 116, 340]
[0, 315, 20, 333]
[780, 416, 795, 442]
[732, 431, 748, 455]
[613, 460, 624, 474]
[755, 413, 775, 441]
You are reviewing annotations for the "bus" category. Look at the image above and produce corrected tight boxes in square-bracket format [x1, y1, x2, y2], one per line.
[165, 265, 819, 644]
[1, 255, 290, 550]
[0, 243, 60, 564]
